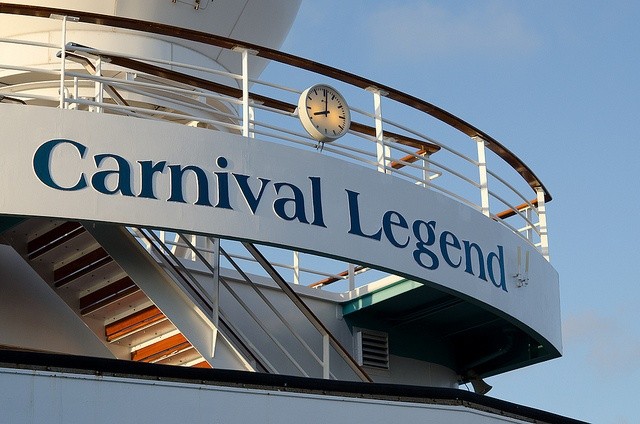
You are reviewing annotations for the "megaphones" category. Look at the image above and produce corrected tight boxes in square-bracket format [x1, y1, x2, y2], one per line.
[471, 374, 492, 394]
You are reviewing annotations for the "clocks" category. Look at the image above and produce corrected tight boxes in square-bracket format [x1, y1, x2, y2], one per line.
[299, 84, 351, 141]
